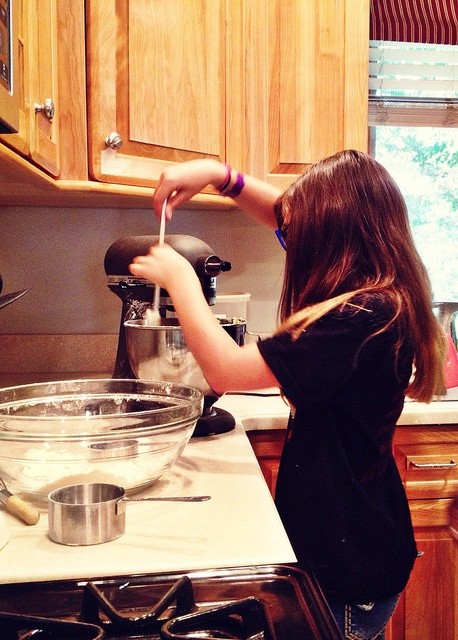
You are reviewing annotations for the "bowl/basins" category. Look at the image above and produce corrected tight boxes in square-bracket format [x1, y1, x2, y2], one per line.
[122, 315, 248, 410]
[0, 378, 205, 501]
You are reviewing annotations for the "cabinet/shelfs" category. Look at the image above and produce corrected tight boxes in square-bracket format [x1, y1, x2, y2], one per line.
[58, 0, 373, 207]
[252, 419, 458, 637]
[1, 0, 57, 208]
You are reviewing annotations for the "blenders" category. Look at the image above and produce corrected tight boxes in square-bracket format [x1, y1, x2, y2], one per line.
[103, 234, 237, 437]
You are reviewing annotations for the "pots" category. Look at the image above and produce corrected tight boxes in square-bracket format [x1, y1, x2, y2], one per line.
[45, 482, 211, 548]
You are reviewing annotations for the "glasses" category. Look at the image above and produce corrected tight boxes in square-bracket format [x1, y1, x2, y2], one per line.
[274, 226, 287, 252]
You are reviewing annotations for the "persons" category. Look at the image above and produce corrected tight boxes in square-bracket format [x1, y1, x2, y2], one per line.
[128, 149, 450, 640]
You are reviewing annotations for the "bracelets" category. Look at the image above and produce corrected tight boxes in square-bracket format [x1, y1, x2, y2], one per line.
[226, 171, 244, 199]
[216, 162, 232, 192]
